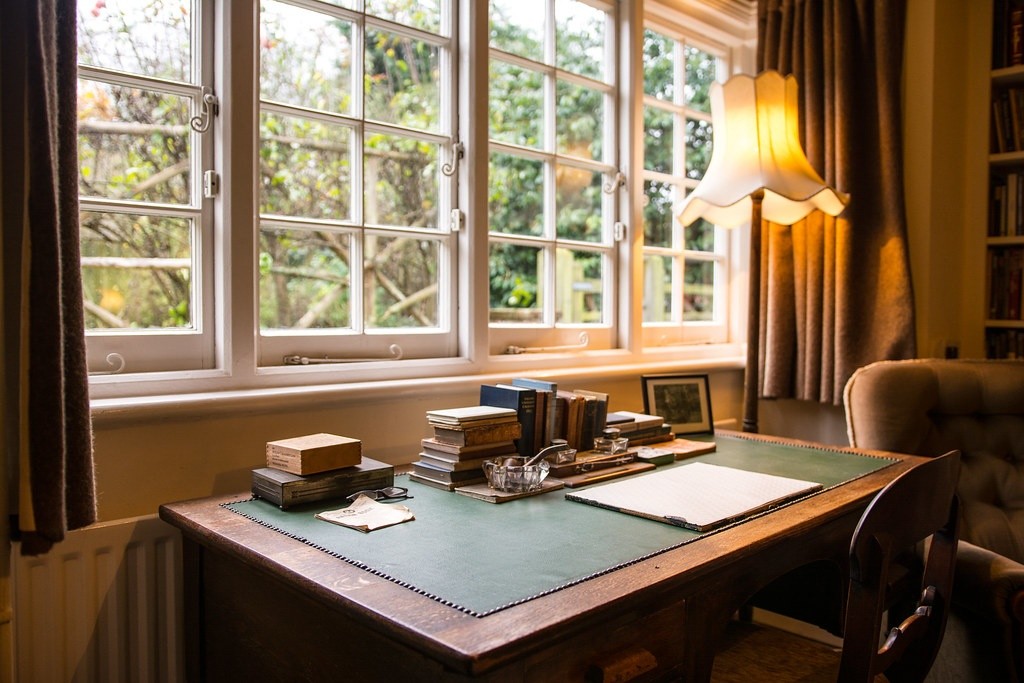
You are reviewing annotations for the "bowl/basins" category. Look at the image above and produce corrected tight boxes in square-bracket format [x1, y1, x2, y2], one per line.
[482, 455, 550, 493]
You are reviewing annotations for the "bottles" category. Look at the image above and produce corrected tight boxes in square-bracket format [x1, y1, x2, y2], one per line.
[594, 428, 629, 454]
[540, 439, 577, 464]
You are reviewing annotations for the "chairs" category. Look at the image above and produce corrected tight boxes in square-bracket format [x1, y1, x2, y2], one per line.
[710, 449, 961, 683]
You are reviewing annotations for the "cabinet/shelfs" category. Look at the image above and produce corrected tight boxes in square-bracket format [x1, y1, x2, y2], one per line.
[981, 0, 1024, 360]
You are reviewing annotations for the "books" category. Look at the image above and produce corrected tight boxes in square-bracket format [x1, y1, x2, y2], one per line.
[987, 250, 1022, 320]
[986, 328, 1024, 361]
[606, 410, 675, 448]
[647, 437, 716, 460]
[992, 88, 1024, 153]
[454, 475, 564, 504]
[407, 405, 521, 491]
[993, 0, 1024, 68]
[988, 173, 1024, 237]
[479, 378, 609, 458]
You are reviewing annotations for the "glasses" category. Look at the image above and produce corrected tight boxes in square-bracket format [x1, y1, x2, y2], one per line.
[345, 487, 414, 504]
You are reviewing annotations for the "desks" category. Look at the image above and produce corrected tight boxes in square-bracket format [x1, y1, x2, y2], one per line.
[159, 427, 937, 683]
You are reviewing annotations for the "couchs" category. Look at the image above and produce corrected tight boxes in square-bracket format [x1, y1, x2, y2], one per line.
[842, 359, 1024, 683]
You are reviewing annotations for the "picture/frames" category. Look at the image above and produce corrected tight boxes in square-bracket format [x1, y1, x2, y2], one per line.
[641, 374, 714, 436]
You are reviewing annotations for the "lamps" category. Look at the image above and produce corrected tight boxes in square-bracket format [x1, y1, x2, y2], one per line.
[669, 70, 851, 434]
